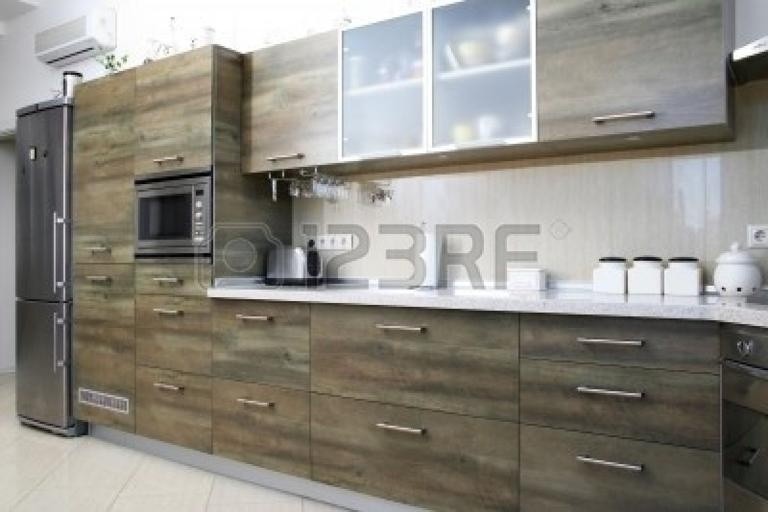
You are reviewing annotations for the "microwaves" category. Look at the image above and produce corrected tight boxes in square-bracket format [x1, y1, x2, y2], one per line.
[133, 175, 212, 258]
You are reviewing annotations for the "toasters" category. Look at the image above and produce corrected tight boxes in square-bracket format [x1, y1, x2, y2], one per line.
[265, 239, 325, 285]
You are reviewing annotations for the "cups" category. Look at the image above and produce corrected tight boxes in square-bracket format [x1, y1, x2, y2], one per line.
[289, 173, 353, 203]
[450, 114, 503, 141]
[61, 72, 82, 98]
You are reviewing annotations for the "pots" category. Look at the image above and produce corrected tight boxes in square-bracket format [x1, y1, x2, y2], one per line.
[713, 241, 763, 297]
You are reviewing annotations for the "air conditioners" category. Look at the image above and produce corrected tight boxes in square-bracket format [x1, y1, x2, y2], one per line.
[33, 9, 118, 69]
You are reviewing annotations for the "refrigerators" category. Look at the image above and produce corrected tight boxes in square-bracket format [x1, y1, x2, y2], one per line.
[13, 98, 88, 438]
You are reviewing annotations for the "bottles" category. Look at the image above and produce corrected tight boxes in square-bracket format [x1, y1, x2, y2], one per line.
[593, 255, 704, 296]
[595, 295, 700, 306]
[410, 36, 423, 78]
[379, 61, 398, 82]
[347, 55, 363, 90]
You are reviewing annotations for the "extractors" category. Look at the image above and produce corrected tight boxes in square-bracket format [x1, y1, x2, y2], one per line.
[730, 35, 768, 85]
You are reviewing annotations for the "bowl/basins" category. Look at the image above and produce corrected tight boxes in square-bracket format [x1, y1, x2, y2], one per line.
[497, 23, 529, 59]
[458, 41, 496, 66]
